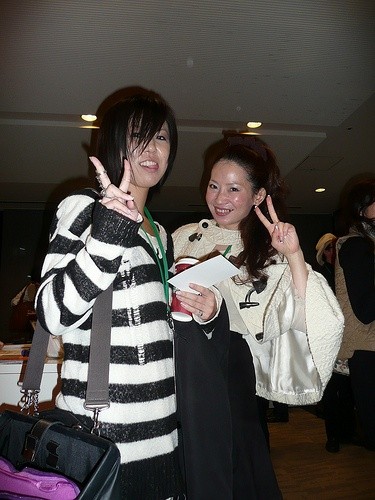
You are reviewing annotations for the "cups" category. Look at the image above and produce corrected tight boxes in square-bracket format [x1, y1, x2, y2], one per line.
[170, 258, 201, 322]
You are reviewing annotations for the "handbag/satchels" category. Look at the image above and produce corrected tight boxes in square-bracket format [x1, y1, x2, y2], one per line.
[0, 409, 121, 500]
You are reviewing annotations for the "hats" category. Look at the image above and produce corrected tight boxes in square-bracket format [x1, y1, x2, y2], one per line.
[315, 233, 337, 267]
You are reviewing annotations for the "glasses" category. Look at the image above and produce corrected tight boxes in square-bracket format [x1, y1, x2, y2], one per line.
[329, 245, 336, 249]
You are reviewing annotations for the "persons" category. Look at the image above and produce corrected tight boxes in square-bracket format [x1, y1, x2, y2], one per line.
[8, 269, 41, 337]
[161, 134, 345, 500]
[314, 233, 364, 453]
[33, 86, 232, 500]
[334, 181, 375, 452]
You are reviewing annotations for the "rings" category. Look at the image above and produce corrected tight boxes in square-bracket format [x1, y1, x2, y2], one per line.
[95, 170, 108, 176]
[199, 310, 203, 318]
[100, 188, 108, 197]
